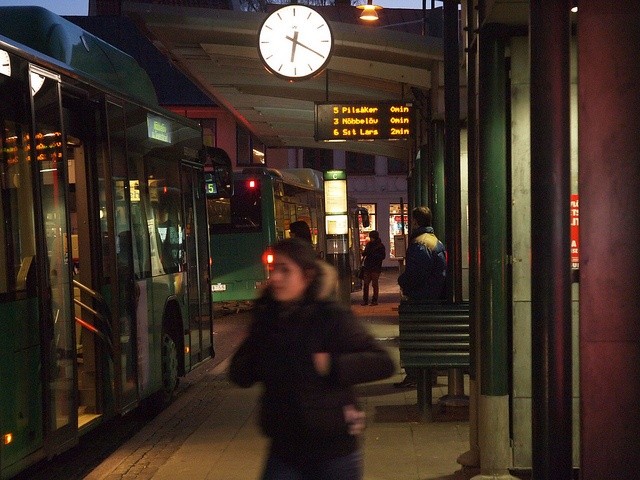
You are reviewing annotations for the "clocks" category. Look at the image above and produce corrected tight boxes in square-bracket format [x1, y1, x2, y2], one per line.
[257, 3, 335, 82]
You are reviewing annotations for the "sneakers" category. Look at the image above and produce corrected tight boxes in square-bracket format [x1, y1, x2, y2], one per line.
[394, 377, 418, 389]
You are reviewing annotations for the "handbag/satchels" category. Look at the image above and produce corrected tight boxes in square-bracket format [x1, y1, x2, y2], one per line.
[359, 265, 366, 279]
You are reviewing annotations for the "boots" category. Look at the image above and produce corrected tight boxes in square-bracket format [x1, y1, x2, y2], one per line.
[371, 296, 378, 305]
[361, 295, 368, 305]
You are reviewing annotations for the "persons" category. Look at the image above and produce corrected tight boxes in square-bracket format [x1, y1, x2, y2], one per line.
[289, 220, 311, 247]
[396, 208, 448, 389]
[228, 238, 396, 480]
[361, 230, 386, 305]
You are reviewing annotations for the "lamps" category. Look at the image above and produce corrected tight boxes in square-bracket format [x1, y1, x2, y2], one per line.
[356, 1, 383, 21]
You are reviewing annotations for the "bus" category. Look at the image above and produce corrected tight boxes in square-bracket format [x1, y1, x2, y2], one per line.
[200, 165, 370, 315]
[1, 7, 233, 475]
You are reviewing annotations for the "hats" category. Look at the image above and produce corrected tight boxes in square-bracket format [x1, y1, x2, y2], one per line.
[369, 230, 379, 237]
